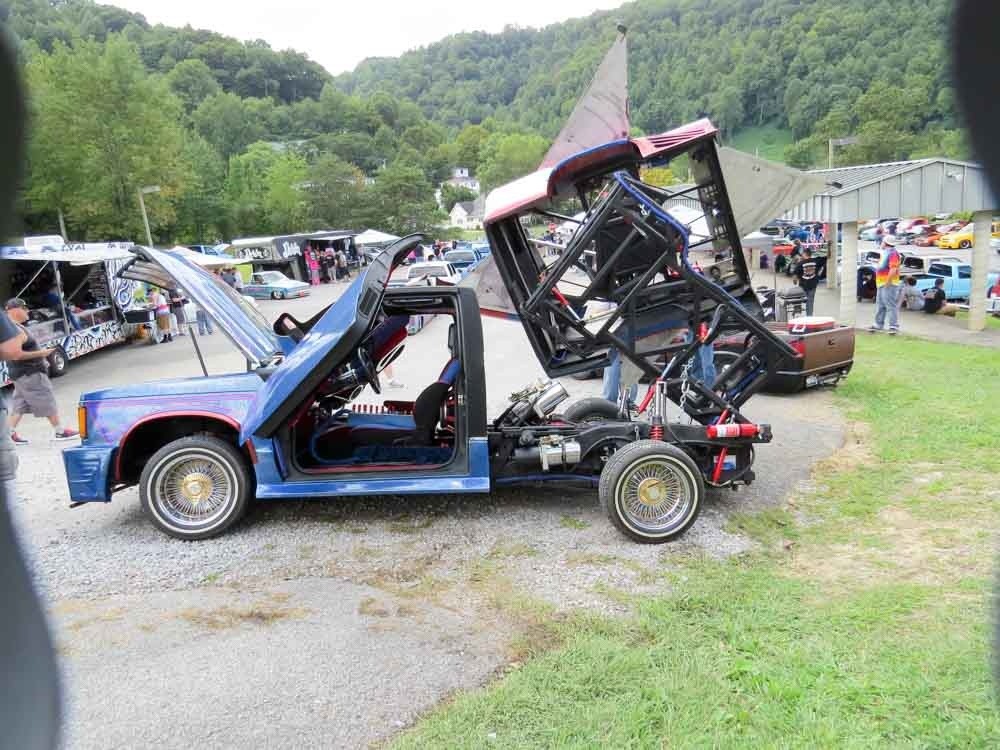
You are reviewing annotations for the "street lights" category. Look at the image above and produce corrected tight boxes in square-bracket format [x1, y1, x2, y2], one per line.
[136, 185, 162, 250]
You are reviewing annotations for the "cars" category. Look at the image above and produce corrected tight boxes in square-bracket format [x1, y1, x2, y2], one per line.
[384, 241, 493, 287]
[234, 270, 312, 301]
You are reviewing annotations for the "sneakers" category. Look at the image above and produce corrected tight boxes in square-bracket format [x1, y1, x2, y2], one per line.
[888, 328, 897, 334]
[56, 428, 80, 440]
[9, 431, 32, 445]
[867, 326, 881, 331]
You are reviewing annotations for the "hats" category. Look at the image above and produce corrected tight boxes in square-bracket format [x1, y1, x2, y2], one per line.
[883, 235, 896, 246]
[5, 298, 30, 310]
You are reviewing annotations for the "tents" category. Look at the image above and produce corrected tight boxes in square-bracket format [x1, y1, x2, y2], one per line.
[231, 229, 360, 273]
[355, 228, 401, 265]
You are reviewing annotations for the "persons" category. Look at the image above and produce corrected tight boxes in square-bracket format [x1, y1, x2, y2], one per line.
[406, 246, 448, 265]
[304, 246, 352, 285]
[147, 268, 244, 344]
[547, 220, 574, 242]
[868, 234, 960, 334]
[775, 226, 823, 318]
[687, 317, 716, 407]
[692, 260, 705, 276]
[0, 297, 80, 480]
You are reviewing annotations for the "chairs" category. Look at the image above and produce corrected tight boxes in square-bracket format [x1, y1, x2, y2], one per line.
[347, 325, 460, 444]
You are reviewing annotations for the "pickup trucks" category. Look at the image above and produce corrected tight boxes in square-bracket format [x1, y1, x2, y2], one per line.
[759, 216, 1000, 304]
[56, 232, 773, 545]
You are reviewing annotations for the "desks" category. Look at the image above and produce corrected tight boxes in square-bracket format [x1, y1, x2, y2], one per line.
[121, 313, 177, 342]
